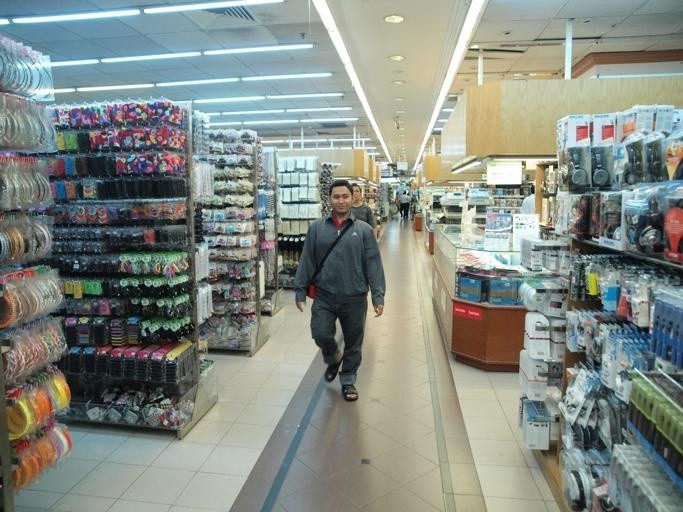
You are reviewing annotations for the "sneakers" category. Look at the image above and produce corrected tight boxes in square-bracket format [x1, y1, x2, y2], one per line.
[342, 384, 358, 401]
[325, 351, 343, 382]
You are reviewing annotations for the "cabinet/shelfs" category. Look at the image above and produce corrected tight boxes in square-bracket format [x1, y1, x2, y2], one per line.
[209, 129, 319, 358]
[530, 230, 683, 512]
[0, 92, 216, 512]
[336, 177, 380, 223]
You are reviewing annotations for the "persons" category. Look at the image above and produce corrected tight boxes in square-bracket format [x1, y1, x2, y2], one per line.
[294, 179, 386, 401]
[522, 185, 536, 215]
[350, 183, 377, 228]
[396, 190, 412, 221]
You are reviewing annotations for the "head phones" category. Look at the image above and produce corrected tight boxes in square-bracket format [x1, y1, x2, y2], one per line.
[563, 141, 664, 250]
[570, 468, 617, 512]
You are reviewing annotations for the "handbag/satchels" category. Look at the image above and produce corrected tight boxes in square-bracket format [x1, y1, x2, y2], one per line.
[307, 283, 316, 298]
